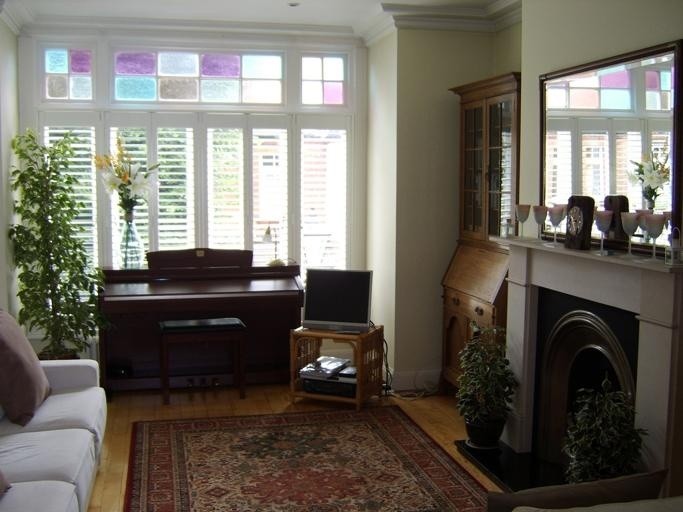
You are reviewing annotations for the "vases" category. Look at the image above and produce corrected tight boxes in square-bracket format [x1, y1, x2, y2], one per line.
[119, 221, 142, 271]
[644, 201, 654, 244]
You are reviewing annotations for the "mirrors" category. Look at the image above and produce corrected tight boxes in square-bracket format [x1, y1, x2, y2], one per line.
[541, 40, 682, 258]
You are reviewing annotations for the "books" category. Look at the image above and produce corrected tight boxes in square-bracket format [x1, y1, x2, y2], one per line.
[300, 355, 351, 381]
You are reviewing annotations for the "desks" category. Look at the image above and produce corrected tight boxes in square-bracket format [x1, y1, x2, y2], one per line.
[290, 326, 384, 413]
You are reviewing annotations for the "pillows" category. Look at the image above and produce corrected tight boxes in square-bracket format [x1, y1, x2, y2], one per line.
[511, 497, 683, 511]
[0, 310, 53, 425]
[489, 466, 669, 512]
[0, 472, 12, 499]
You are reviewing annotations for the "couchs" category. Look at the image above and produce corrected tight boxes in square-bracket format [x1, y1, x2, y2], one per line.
[0, 359, 107, 512]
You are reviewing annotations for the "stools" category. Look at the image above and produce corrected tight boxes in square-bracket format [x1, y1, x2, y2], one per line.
[159, 319, 248, 405]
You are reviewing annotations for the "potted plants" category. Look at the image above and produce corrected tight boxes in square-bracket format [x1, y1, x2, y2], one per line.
[6, 128, 114, 360]
[455, 321, 519, 447]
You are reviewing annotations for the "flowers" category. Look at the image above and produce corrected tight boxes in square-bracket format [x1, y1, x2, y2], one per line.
[99, 139, 161, 271]
[629, 147, 671, 201]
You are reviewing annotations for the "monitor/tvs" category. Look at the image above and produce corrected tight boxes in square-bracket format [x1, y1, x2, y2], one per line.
[302, 268, 374, 335]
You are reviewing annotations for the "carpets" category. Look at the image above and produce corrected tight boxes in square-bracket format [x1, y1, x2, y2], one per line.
[122, 405, 491, 512]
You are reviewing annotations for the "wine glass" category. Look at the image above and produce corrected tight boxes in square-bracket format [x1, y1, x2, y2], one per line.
[531, 206, 548, 243]
[514, 204, 530, 242]
[663, 211, 672, 231]
[554, 204, 568, 234]
[595, 210, 613, 256]
[636, 209, 654, 243]
[639, 214, 666, 264]
[544, 206, 563, 247]
[619, 213, 640, 259]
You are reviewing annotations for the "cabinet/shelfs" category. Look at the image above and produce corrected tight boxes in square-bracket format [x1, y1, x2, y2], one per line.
[440, 71, 521, 403]
